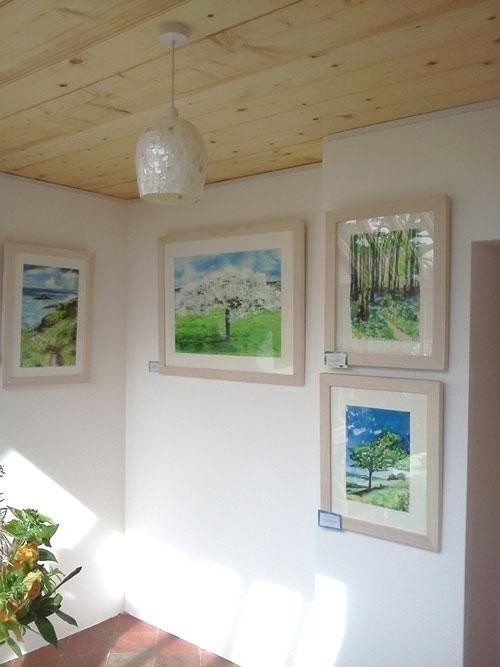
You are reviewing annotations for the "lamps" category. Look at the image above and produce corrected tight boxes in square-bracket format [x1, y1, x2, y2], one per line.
[133, 25, 207, 209]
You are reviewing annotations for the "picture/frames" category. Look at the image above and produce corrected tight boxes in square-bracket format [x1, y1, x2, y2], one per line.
[149, 217, 307, 389]
[320, 193, 449, 374]
[2, 235, 98, 387]
[318, 370, 446, 551]
